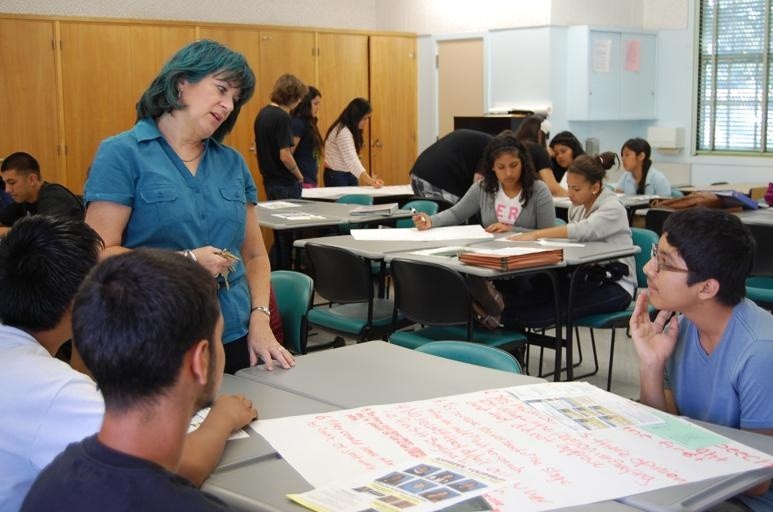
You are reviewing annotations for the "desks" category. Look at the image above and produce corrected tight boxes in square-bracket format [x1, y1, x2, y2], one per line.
[293, 219, 517, 345]
[549, 192, 655, 228]
[196, 434, 648, 510]
[232, 339, 718, 508]
[244, 197, 416, 269]
[636, 204, 773, 279]
[387, 234, 641, 382]
[169, 373, 350, 478]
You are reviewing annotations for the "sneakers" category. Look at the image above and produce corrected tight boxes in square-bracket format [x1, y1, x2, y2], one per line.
[466, 273, 505, 315]
[471, 299, 505, 331]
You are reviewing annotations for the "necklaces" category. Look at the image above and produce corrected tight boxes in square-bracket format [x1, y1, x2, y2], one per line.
[181, 146, 206, 163]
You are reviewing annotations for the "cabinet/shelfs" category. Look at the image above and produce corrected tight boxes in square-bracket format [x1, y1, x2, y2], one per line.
[199, 21, 318, 274]
[57, 14, 199, 198]
[566, 25, 660, 122]
[0, 13, 66, 191]
[318, 27, 419, 190]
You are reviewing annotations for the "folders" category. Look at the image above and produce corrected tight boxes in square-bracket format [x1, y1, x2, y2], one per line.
[457, 240, 563, 272]
[351, 202, 399, 216]
[699, 189, 758, 210]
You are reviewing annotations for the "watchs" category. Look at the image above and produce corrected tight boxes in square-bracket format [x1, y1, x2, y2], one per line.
[249, 304, 273, 317]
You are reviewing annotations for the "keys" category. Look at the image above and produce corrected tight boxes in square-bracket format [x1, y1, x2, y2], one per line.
[219, 250, 240, 292]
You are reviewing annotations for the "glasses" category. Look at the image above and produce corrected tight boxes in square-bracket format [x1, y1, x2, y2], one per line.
[649, 242, 689, 273]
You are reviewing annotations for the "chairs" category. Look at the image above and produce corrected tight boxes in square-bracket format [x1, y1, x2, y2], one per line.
[262, 270, 315, 359]
[389, 259, 530, 365]
[396, 199, 439, 231]
[413, 341, 534, 378]
[337, 193, 373, 207]
[571, 228, 668, 395]
[303, 242, 403, 356]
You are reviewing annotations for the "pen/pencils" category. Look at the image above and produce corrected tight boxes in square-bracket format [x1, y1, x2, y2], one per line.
[410, 206, 428, 226]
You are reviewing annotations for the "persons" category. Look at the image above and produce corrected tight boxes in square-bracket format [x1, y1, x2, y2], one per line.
[614, 139, 672, 199]
[630, 207, 773, 511]
[549, 131, 585, 188]
[253, 71, 305, 271]
[408, 130, 517, 206]
[0, 213, 259, 511]
[468, 152, 639, 331]
[18, 249, 233, 511]
[323, 97, 384, 189]
[412, 130, 556, 233]
[290, 84, 323, 189]
[0, 150, 83, 237]
[516, 115, 571, 199]
[84, 39, 297, 376]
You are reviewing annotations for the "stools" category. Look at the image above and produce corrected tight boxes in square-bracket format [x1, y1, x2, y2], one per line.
[745, 275, 773, 319]
